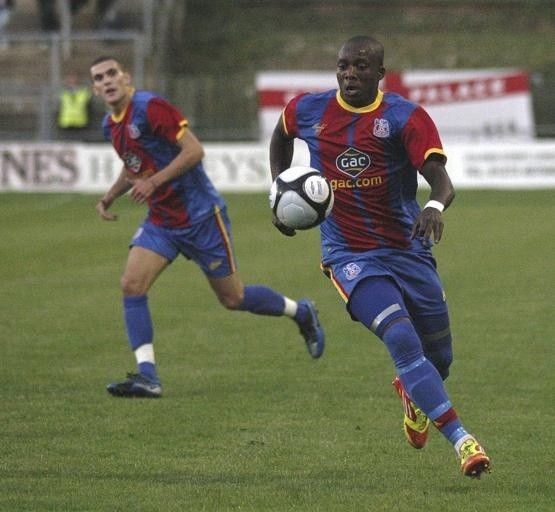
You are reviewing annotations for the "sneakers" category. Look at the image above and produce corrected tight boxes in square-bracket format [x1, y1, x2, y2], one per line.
[457, 438, 492, 480]
[106, 372, 163, 397]
[294, 298, 325, 358]
[389, 376, 429, 448]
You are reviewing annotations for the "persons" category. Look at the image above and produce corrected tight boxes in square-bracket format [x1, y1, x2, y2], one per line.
[50, 69, 95, 140]
[89, 54, 325, 396]
[270, 37, 488, 477]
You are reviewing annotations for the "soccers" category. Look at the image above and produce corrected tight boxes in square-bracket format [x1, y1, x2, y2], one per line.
[269, 164, 335, 231]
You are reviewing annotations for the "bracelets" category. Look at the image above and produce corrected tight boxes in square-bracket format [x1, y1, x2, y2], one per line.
[423, 200, 446, 212]
